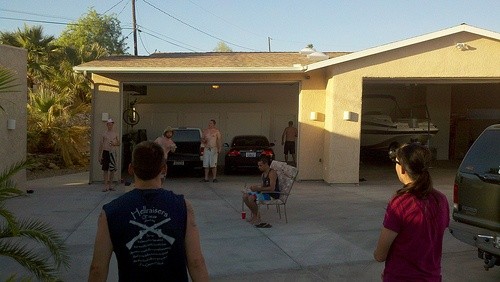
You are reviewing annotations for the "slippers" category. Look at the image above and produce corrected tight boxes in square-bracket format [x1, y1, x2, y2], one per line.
[257, 223, 272, 228]
[204, 178, 217, 182]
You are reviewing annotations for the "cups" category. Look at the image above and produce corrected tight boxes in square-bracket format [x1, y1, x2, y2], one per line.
[242, 212, 246, 220]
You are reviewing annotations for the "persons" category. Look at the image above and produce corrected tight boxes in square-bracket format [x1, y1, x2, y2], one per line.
[282, 121, 298, 167]
[152, 127, 178, 185]
[374, 144, 450, 282]
[88, 141, 210, 282]
[99, 119, 121, 192]
[244, 154, 279, 223]
[201, 120, 222, 182]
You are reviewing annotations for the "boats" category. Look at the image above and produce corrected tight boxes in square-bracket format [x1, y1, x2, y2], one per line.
[359, 94, 439, 164]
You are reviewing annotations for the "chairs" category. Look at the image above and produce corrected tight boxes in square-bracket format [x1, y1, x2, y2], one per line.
[241, 160, 299, 224]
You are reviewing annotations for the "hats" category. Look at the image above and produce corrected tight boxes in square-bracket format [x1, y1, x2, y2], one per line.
[107, 119, 115, 125]
[163, 126, 174, 134]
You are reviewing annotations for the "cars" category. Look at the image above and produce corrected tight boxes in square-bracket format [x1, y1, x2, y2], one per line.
[448, 123, 500, 271]
[224, 134, 276, 175]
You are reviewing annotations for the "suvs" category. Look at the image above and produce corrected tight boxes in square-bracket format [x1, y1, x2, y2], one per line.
[166, 127, 205, 177]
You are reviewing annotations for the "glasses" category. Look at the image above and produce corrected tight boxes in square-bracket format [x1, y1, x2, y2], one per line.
[389, 152, 401, 166]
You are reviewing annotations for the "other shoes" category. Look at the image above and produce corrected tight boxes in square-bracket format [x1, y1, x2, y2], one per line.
[102, 187, 115, 192]
